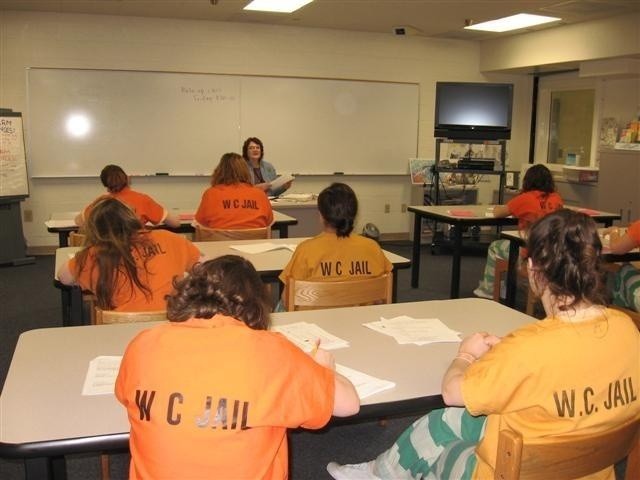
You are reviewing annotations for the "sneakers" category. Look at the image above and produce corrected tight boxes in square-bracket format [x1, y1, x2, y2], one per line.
[326, 460, 382, 480]
[473, 288, 493, 299]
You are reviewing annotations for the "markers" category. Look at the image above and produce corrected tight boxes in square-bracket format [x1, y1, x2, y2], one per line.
[155, 173, 169, 175]
[334, 172, 344, 175]
[28, 68, 418, 177]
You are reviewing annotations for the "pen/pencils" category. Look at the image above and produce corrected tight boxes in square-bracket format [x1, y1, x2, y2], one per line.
[270, 197, 280, 201]
[311, 338, 321, 358]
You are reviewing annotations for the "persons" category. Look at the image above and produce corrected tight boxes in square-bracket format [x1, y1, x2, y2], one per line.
[242, 137, 292, 196]
[326, 208, 640, 480]
[592, 220, 640, 313]
[473, 164, 564, 300]
[114, 255, 360, 480]
[58, 198, 207, 313]
[76, 165, 180, 227]
[191, 152, 276, 242]
[273, 183, 394, 312]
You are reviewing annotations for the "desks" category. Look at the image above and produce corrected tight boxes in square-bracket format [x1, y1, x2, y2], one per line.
[53, 236, 411, 325]
[407, 203, 622, 299]
[44, 209, 298, 328]
[0, 296, 545, 480]
[500, 227, 640, 308]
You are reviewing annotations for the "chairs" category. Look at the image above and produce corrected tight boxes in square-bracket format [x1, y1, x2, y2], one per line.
[94, 306, 168, 325]
[495, 414, 640, 480]
[191, 218, 272, 242]
[69, 229, 86, 247]
[286, 271, 393, 311]
[493, 258, 534, 316]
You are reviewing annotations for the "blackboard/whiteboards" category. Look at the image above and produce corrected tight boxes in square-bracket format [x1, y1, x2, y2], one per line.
[1, 113, 29, 195]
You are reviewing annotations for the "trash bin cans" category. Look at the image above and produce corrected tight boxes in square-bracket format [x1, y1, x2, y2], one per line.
[362, 223, 380, 243]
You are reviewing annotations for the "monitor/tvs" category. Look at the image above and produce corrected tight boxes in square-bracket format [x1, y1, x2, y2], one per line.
[434, 81, 514, 143]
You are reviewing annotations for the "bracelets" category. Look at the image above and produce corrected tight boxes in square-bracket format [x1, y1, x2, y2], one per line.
[455, 352, 475, 365]
[609, 228, 620, 237]
